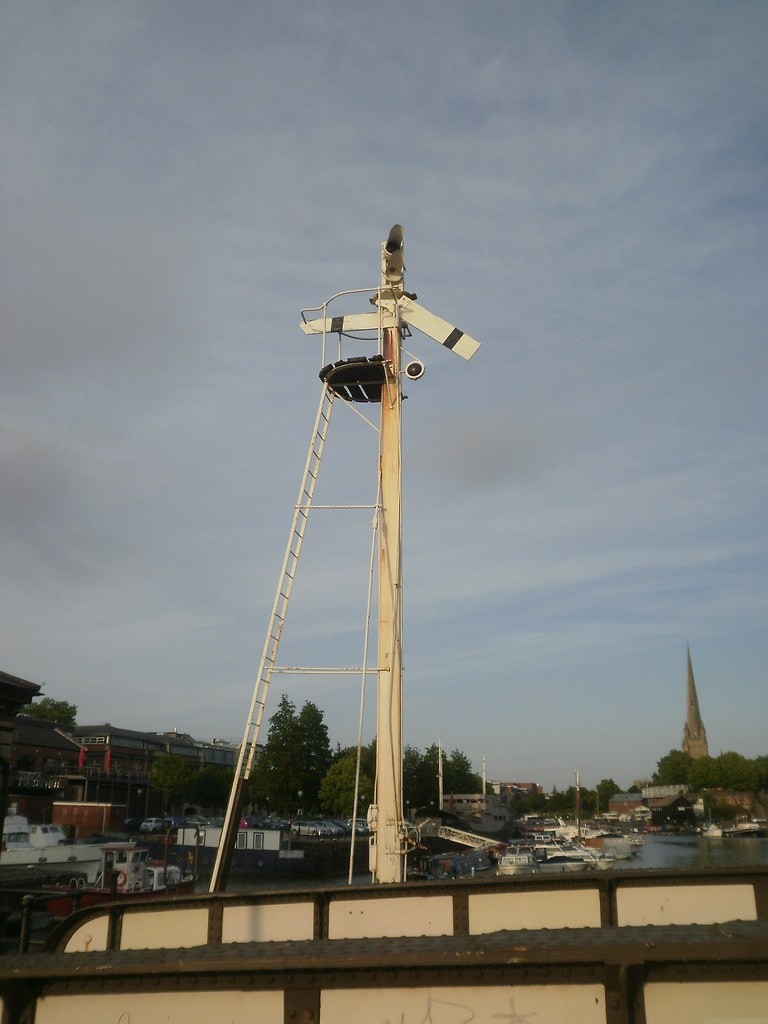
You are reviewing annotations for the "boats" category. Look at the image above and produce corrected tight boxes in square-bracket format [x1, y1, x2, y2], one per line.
[0, 800, 201, 923]
[405, 813, 647, 880]
[703, 824, 721, 837]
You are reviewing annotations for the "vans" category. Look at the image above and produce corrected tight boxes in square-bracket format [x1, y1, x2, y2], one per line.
[139, 818, 164, 834]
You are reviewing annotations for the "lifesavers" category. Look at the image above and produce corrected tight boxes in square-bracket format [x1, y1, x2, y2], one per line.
[116, 871, 127, 888]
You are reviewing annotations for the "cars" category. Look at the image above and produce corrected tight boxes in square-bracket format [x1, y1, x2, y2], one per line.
[208, 814, 289, 829]
[313, 820, 369, 836]
[290, 820, 330, 837]
